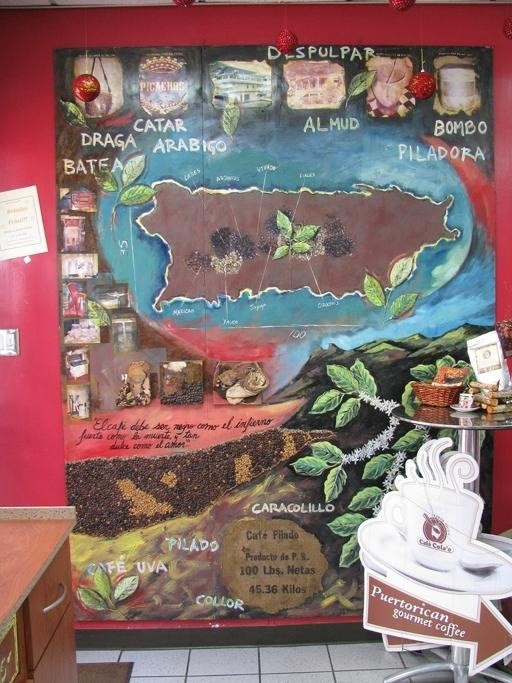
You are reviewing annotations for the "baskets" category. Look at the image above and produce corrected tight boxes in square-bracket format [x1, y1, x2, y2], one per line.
[410, 381, 463, 407]
[412, 404, 459, 425]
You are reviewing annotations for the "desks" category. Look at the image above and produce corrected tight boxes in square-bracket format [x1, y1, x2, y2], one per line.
[383, 404, 512, 683]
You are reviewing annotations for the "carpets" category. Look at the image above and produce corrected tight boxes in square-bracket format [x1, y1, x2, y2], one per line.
[76, 662, 134, 683]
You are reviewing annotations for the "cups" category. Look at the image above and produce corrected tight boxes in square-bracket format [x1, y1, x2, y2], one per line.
[369, 66, 408, 108]
[459, 394, 473, 408]
[458, 417, 473, 426]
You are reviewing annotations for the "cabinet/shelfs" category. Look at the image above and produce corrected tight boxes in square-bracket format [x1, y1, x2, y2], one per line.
[0, 519, 79, 683]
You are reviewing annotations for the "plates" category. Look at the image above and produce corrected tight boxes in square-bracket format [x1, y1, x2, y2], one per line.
[450, 403, 481, 413]
[450, 412, 481, 420]
